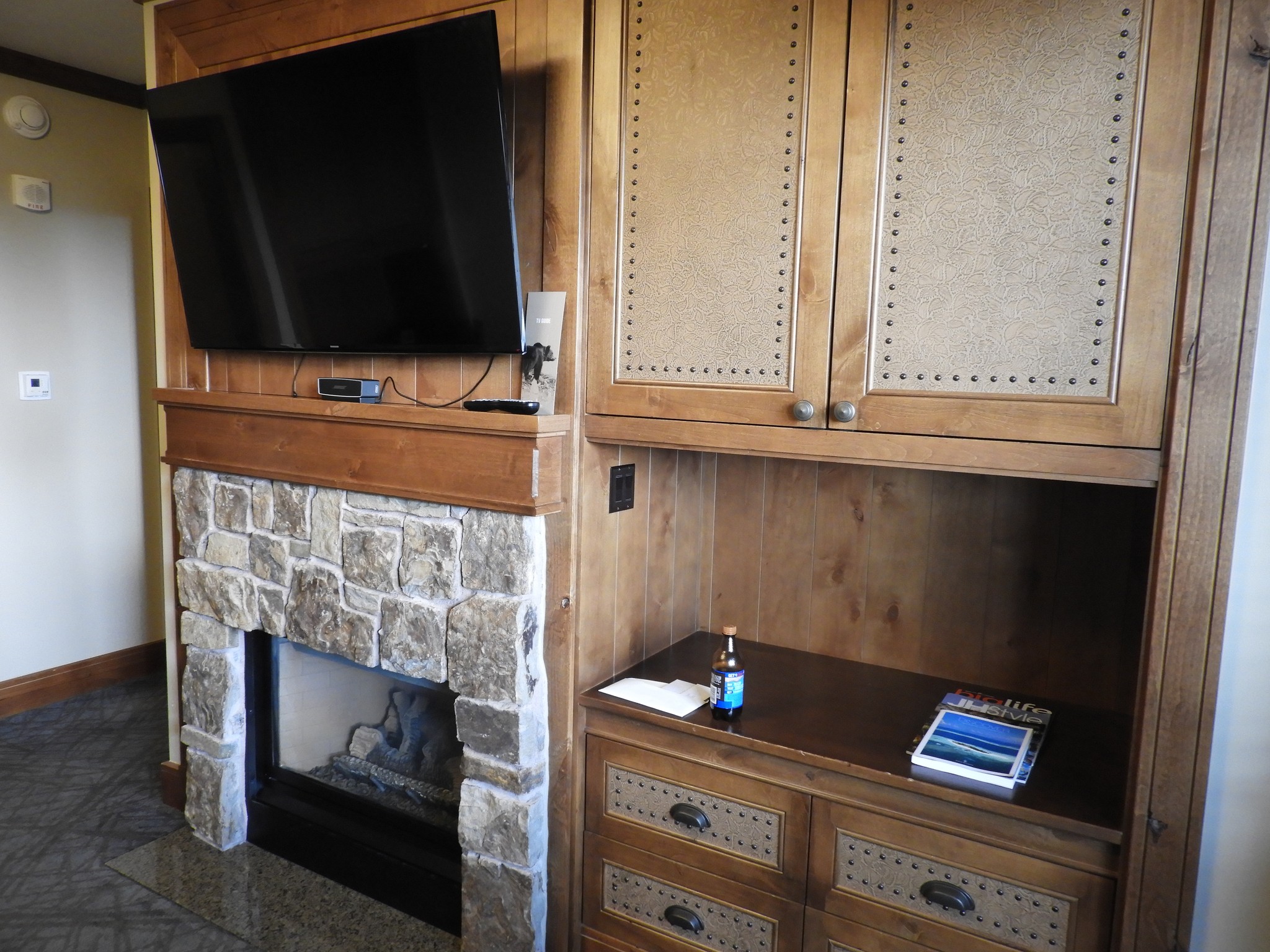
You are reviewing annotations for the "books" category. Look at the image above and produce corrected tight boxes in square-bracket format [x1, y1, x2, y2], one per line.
[906, 687, 1058, 783]
[911, 709, 1034, 789]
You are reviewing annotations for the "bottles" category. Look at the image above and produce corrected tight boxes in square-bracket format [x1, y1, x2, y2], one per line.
[710, 625, 745, 721]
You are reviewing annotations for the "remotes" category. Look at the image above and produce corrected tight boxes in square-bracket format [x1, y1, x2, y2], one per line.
[464, 399, 539, 415]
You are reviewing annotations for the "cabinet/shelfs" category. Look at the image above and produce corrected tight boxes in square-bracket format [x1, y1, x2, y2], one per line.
[554, 0, 1270, 952]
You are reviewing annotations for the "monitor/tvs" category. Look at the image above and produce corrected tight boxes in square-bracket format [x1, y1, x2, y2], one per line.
[145, 8, 527, 359]
